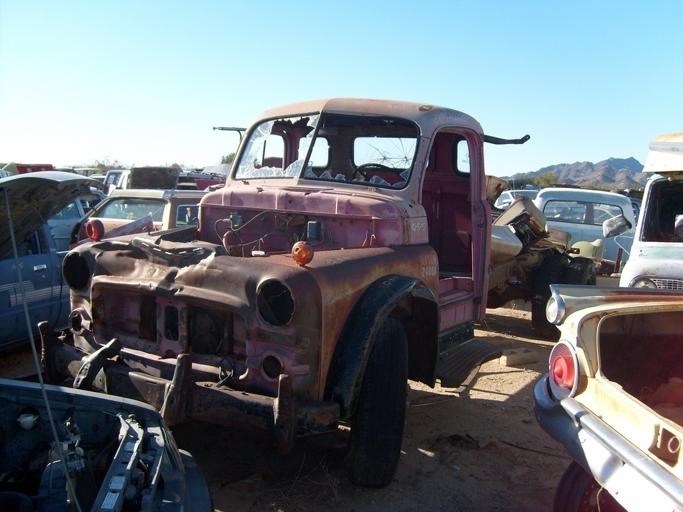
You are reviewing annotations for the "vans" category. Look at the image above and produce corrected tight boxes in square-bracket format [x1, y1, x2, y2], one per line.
[617, 135, 683, 292]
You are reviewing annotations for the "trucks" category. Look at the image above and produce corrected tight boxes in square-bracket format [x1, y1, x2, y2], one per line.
[35, 97, 608, 491]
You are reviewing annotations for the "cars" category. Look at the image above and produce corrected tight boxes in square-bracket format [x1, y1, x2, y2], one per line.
[529, 281, 683, 490]
[1, 378, 214, 512]
[492, 178, 642, 277]
